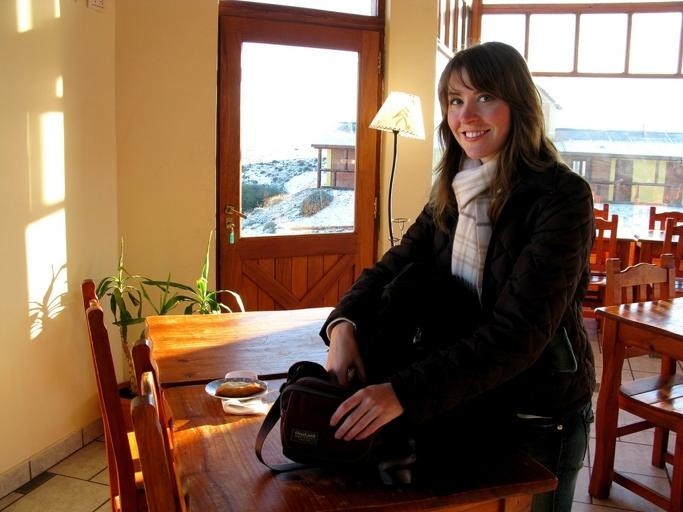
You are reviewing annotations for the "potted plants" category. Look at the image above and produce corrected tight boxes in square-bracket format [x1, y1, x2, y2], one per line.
[98, 232, 139, 431]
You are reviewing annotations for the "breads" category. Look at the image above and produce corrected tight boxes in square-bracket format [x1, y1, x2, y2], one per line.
[217, 381, 264, 397]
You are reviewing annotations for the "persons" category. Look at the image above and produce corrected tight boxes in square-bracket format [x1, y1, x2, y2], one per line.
[316, 39, 597, 511]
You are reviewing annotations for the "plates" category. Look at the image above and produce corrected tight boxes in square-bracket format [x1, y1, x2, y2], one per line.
[205, 377, 268, 401]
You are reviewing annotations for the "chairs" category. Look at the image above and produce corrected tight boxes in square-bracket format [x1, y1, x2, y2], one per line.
[79, 278, 178, 512]
[579, 202, 683, 511]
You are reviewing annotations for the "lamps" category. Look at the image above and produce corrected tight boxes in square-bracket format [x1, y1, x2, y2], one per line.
[369, 90, 424, 250]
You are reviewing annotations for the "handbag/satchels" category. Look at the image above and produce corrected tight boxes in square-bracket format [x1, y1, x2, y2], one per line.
[276, 361, 422, 501]
